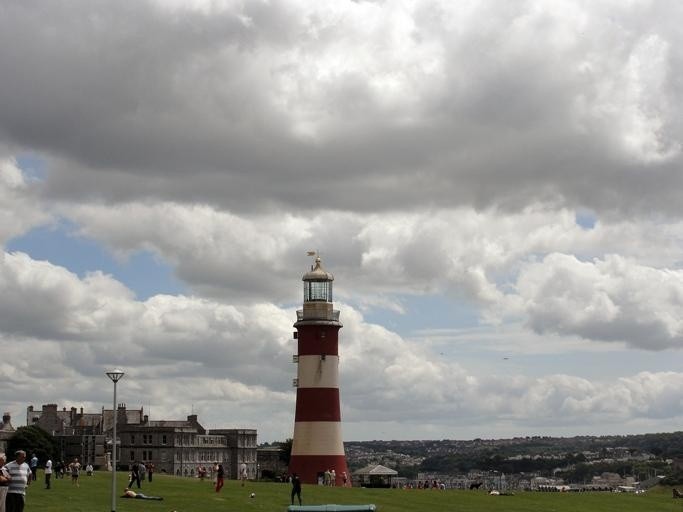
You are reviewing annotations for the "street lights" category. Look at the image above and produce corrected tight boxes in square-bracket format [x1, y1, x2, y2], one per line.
[105, 366, 125, 512]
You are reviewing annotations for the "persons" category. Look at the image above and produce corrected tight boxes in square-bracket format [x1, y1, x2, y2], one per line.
[216, 465, 224, 491]
[292, 473, 301, 505]
[0, 450, 93, 512]
[120, 488, 163, 500]
[241, 469, 247, 486]
[213, 462, 219, 484]
[127, 460, 155, 488]
[392, 480, 438, 489]
[318, 469, 347, 486]
